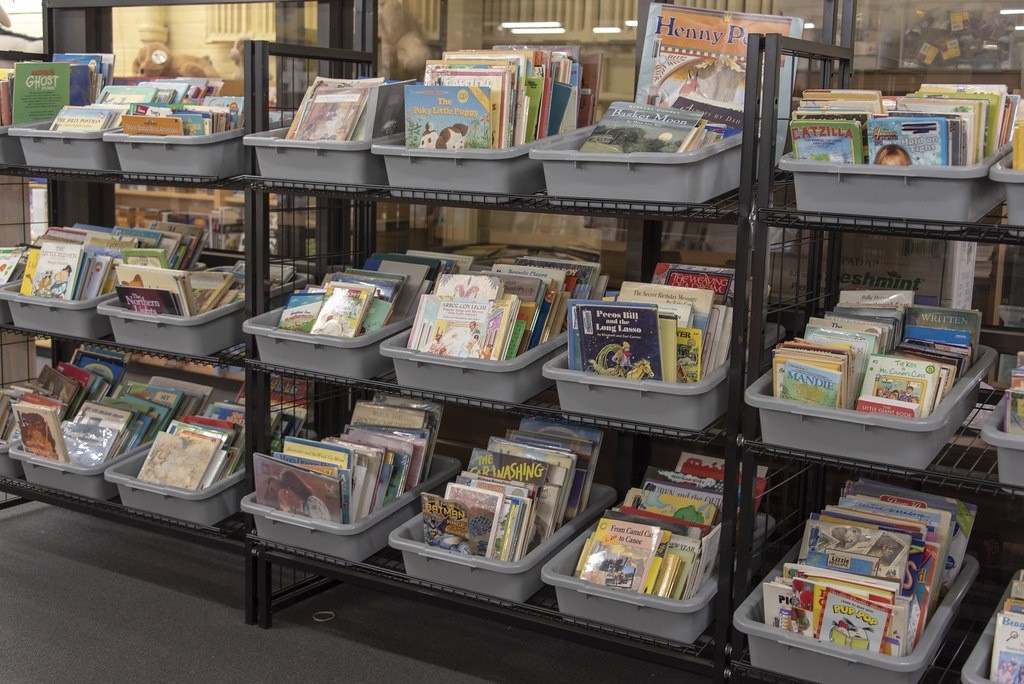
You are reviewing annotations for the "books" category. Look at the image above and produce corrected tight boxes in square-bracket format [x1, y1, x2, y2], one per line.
[1003, 351, 1024, 435]
[989, 579, 1024, 684]
[278, 249, 736, 385]
[286, 44, 604, 149]
[572, 451, 768, 601]
[788, 83, 1024, 170]
[421, 417, 605, 566]
[0, 220, 296, 317]
[771, 290, 982, 417]
[249, 393, 444, 526]
[762, 477, 977, 656]
[0, 53, 244, 136]
[0, 344, 313, 491]
[580, 2, 803, 153]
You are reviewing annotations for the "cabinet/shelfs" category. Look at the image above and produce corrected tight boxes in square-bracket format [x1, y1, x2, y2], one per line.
[254, 0, 838, 684]
[0, 0, 363, 622]
[735, 0, 1024, 684]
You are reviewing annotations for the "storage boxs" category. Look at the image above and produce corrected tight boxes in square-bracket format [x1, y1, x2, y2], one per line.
[0, 119, 1022, 683]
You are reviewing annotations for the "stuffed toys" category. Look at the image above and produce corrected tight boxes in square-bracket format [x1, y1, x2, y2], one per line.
[229, 38, 244, 80]
[132, 43, 219, 78]
